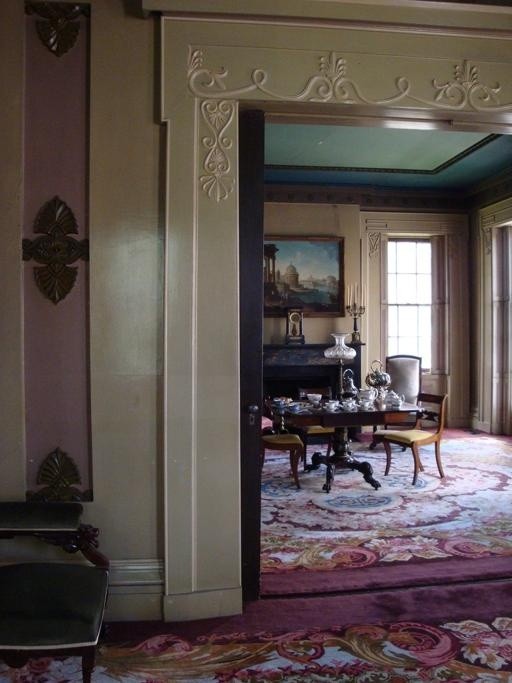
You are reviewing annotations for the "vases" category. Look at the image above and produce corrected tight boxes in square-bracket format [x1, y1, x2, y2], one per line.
[324, 333, 356, 359]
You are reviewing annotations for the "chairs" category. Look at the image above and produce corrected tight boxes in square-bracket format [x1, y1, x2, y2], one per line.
[0, 500, 110, 683]
[368, 355, 422, 451]
[372, 390, 448, 485]
[262, 434, 304, 490]
[298, 386, 335, 465]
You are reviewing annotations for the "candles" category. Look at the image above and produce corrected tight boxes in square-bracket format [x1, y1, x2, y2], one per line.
[346, 282, 368, 307]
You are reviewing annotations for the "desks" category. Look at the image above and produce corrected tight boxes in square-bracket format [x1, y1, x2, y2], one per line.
[264, 398, 424, 493]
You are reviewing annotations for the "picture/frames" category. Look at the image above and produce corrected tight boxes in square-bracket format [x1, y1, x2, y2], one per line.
[263, 235, 344, 318]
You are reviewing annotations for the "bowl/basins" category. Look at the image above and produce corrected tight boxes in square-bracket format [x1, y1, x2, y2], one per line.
[307, 393, 323, 404]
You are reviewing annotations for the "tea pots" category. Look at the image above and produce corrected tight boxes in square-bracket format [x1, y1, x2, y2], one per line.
[356, 389, 378, 399]
[366, 360, 405, 408]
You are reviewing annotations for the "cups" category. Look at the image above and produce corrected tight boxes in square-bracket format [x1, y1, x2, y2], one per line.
[325, 400, 370, 408]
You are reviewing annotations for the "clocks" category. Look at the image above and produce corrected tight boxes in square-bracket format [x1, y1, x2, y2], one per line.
[287, 307, 305, 345]
